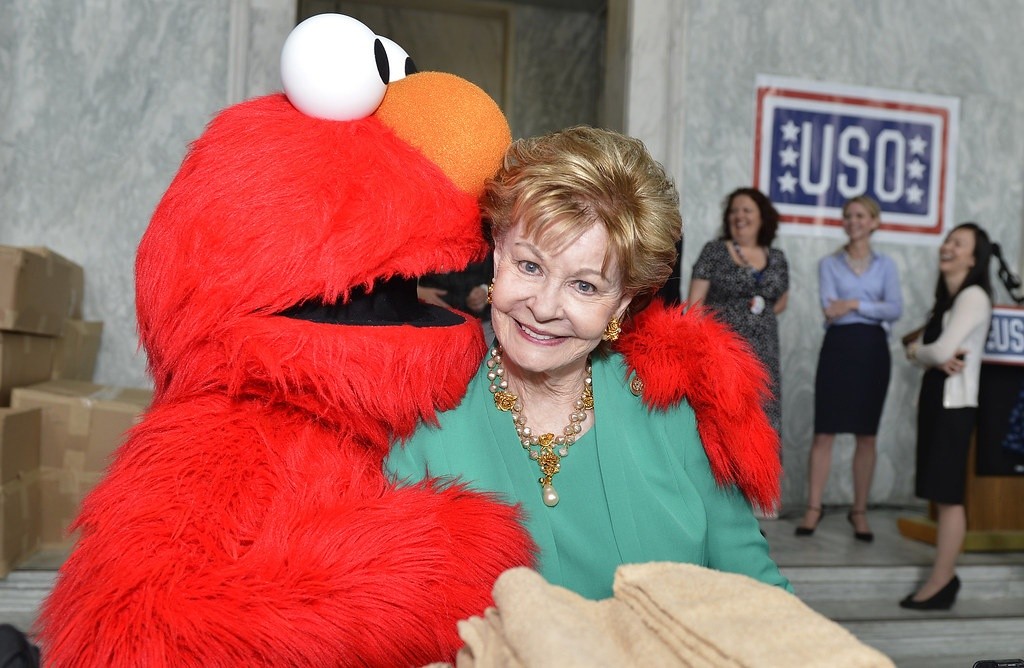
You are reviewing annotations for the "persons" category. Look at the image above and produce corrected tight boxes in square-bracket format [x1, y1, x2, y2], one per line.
[793, 196, 903, 544]
[687, 187, 791, 536]
[898, 223, 993, 610]
[383, 126, 796, 595]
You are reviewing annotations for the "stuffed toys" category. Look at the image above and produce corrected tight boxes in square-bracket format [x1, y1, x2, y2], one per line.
[26, 14, 780, 668]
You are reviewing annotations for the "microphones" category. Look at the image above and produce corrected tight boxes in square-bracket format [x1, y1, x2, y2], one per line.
[991, 243, 1011, 277]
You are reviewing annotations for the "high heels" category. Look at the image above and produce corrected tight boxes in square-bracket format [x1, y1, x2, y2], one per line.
[794, 506, 825, 535]
[848, 508, 876, 541]
[898, 577, 961, 611]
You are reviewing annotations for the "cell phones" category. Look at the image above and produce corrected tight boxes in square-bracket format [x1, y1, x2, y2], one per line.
[942, 354, 965, 380]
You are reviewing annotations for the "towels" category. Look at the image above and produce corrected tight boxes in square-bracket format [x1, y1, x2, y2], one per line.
[455, 558, 899, 667]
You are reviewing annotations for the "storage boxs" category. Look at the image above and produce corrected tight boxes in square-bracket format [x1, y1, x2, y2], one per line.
[0, 239, 153, 583]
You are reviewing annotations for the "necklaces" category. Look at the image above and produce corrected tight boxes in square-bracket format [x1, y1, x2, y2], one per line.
[485, 347, 594, 505]
